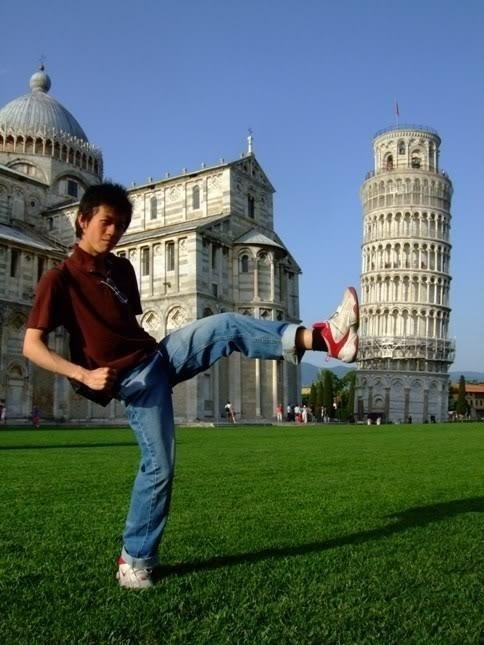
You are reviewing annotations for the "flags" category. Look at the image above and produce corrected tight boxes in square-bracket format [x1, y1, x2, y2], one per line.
[394, 101, 399, 115]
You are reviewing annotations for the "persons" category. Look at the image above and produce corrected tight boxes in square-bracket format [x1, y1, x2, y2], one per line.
[0, 405, 9, 425]
[224, 396, 331, 425]
[29, 401, 42, 430]
[22, 177, 360, 587]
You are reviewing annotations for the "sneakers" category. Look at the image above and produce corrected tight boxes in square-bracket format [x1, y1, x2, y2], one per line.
[115, 555, 154, 590]
[313, 287, 360, 364]
